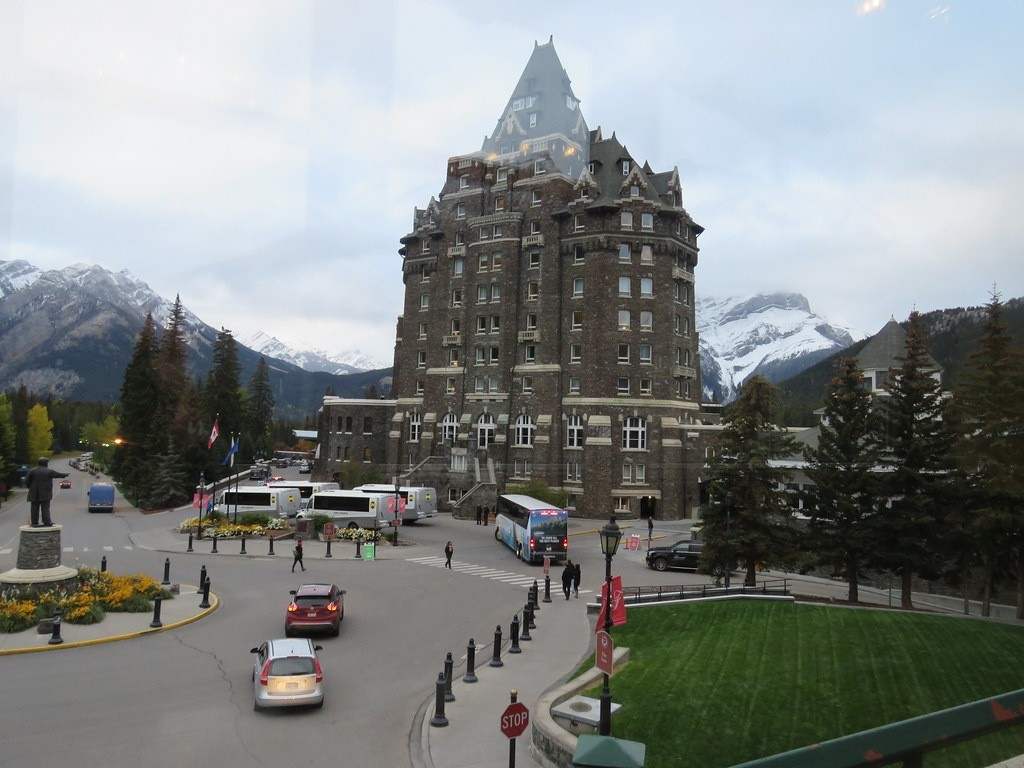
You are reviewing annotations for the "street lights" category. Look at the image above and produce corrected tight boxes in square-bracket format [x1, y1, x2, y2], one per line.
[197, 477, 206, 540]
[723, 492, 736, 589]
[394, 479, 401, 545]
[596, 509, 626, 737]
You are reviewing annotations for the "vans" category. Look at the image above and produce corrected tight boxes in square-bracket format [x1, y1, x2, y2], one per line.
[268, 480, 341, 510]
[352, 484, 439, 523]
[87, 482, 115, 512]
[210, 486, 302, 523]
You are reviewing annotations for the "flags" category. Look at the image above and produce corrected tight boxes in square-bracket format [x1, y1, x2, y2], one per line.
[208, 419, 219, 448]
[231, 437, 235, 467]
[222, 439, 239, 465]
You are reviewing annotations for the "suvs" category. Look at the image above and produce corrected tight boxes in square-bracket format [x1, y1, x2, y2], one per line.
[646, 539, 727, 577]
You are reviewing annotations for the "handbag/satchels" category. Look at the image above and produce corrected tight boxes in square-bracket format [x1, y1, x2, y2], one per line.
[293, 548, 300, 556]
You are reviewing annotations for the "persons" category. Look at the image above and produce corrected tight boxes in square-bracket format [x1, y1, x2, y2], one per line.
[648, 517, 653, 539]
[477, 504, 482, 525]
[562, 560, 581, 600]
[484, 504, 489, 526]
[24, 457, 70, 525]
[206, 500, 212, 514]
[445, 541, 453, 569]
[292, 540, 306, 572]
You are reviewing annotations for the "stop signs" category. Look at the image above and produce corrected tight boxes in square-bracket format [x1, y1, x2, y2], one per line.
[500, 701, 530, 739]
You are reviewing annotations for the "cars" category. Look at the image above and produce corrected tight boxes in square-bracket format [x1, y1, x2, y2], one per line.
[294, 490, 403, 530]
[249, 458, 316, 482]
[68, 451, 101, 475]
[59, 480, 72, 489]
[284, 583, 346, 637]
[251, 637, 325, 710]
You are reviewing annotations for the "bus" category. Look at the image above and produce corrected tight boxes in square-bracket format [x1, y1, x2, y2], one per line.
[492, 494, 569, 564]
